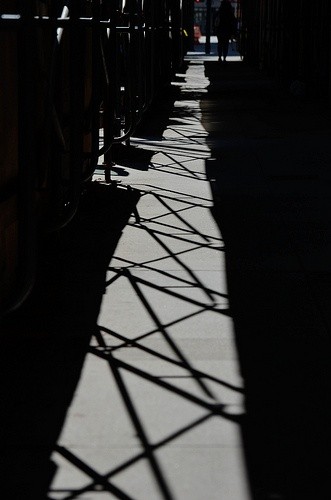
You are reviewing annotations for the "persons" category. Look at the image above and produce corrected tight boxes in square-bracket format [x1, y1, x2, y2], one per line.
[214, 1, 238, 65]
